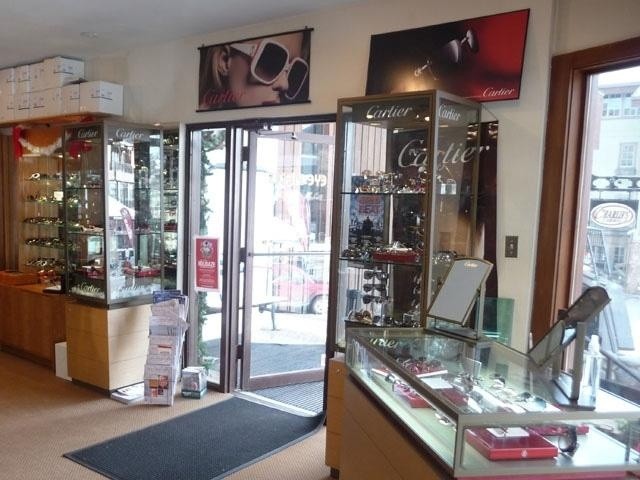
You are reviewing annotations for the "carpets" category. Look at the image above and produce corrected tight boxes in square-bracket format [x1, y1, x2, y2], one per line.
[59, 392, 329, 480]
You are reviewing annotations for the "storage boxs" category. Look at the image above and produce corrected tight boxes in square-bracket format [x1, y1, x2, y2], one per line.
[1, 55, 125, 122]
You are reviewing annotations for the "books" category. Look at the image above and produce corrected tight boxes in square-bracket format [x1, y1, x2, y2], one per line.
[144, 289, 206, 402]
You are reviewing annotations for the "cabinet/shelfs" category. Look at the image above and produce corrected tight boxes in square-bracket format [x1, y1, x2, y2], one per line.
[65, 123, 168, 398]
[0, 128, 67, 374]
[324, 86, 485, 478]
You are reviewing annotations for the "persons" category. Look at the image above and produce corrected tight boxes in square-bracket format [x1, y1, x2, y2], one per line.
[196, 31, 308, 107]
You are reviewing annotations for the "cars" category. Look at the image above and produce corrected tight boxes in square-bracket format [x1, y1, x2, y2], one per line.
[262, 262, 328, 315]
[583, 272, 639, 392]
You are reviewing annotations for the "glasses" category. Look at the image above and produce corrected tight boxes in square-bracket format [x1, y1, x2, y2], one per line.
[413, 27, 479, 81]
[230, 38, 309, 100]
